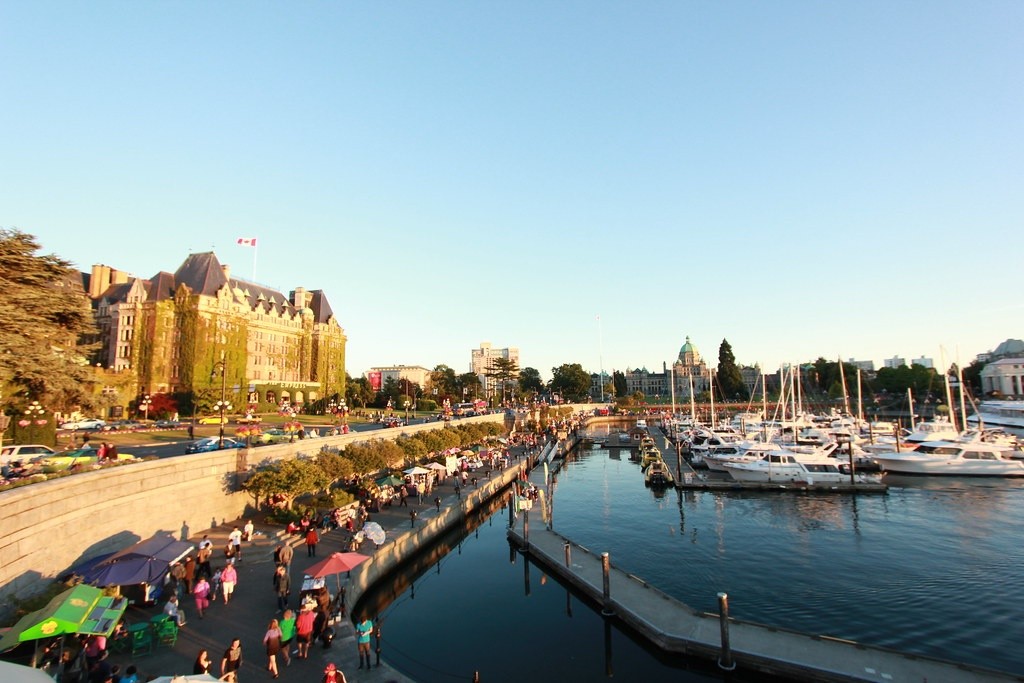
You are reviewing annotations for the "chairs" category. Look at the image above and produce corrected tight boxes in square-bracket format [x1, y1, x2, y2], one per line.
[108, 610, 180, 660]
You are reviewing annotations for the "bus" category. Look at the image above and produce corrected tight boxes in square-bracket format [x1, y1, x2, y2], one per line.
[456, 403, 487, 417]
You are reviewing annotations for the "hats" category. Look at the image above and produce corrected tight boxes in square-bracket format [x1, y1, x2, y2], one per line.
[324, 663, 335, 673]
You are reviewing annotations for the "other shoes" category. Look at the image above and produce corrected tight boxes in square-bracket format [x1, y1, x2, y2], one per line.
[304, 655, 309, 660]
[357, 665, 363, 671]
[286, 659, 291, 667]
[266, 666, 271, 672]
[297, 655, 303, 658]
[368, 665, 372, 670]
[273, 674, 279, 681]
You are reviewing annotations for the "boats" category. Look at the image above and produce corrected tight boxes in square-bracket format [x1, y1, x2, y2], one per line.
[639, 437, 673, 485]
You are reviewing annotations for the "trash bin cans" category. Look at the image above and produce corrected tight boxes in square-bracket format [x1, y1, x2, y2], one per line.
[684, 473, 692, 485]
[383, 418, 400, 428]
[500, 459, 507, 468]
[557, 429, 567, 441]
[413, 483, 418, 497]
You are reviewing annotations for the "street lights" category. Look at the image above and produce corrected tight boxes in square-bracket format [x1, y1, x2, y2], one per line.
[214, 401, 232, 450]
[210, 355, 225, 450]
[142, 395, 151, 420]
[24, 401, 44, 445]
[290, 413, 296, 444]
[403, 401, 410, 426]
[414, 387, 425, 419]
[338, 398, 346, 425]
[246, 415, 252, 449]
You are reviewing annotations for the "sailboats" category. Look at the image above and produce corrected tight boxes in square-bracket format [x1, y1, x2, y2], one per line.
[664, 361, 1024, 483]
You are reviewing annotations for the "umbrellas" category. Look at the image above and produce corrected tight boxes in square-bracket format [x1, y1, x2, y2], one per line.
[304, 551, 370, 591]
[146, 673, 227, 683]
[362, 521, 385, 545]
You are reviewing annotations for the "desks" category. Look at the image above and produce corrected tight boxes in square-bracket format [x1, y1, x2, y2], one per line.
[150, 613, 169, 630]
[127, 622, 148, 639]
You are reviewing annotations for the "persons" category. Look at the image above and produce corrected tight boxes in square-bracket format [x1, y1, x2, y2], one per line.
[321, 662, 347, 683]
[305, 527, 320, 558]
[263, 618, 282, 679]
[193, 648, 213, 675]
[221, 636, 243, 683]
[353, 394, 596, 508]
[279, 540, 294, 594]
[297, 606, 318, 662]
[279, 609, 296, 666]
[298, 405, 352, 440]
[35, 633, 140, 683]
[82, 431, 118, 462]
[356, 616, 373, 670]
[164, 520, 254, 628]
[273, 563, 291, 611]
[317, 586, 332, 609]
[312, 605, 329, 649]
[285, 503, 368, 551]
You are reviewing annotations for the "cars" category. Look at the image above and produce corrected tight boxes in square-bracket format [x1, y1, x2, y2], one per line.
[235, 415, 263, 425]
[185, 436, 247, 455]
[199, 415, 229, 425]
[250, 427, 357, 447]
[1, 445, 136, 478]
[60, 418, 182, 431]
